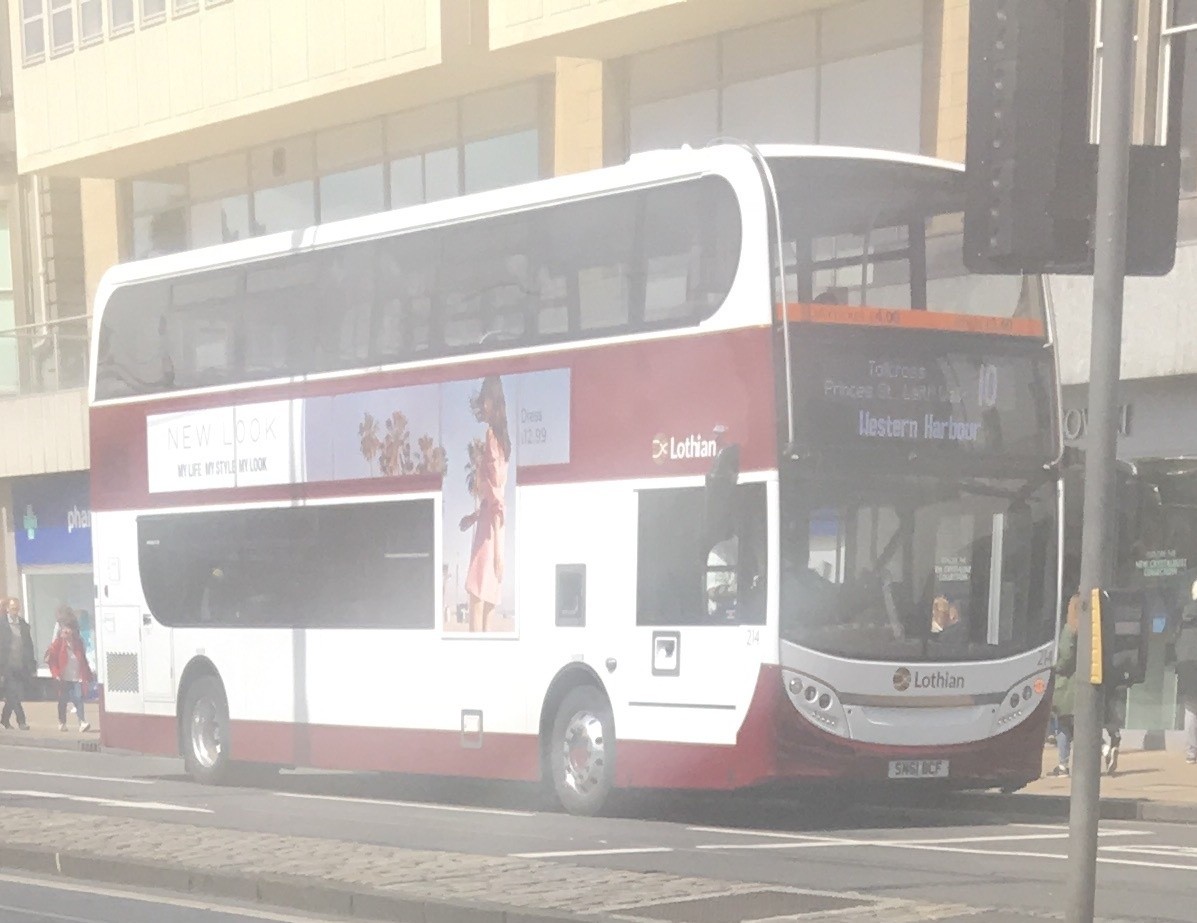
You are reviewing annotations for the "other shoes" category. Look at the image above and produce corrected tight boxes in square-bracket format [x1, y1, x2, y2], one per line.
[79, 721, 92, 734]
[19, 723, 32, 730]
[0, 717, 15, 730]
[57, 724, 69, 731]
[1186, 757, 1195, 764]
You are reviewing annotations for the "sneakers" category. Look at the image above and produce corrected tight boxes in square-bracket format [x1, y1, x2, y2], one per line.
[1047, 765, 1071, 779]
[1104, 744, 1118, 776]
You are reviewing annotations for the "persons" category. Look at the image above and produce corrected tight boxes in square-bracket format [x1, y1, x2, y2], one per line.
[49, 607, 93, 731]
[708, 509, 764, 618]
[1046, 591, 1120, 777]
[931, 597, 967, 633]
[0, 598, 35, 730]
[458, 377, 512, 632]
[1166, 580, 1197, 762]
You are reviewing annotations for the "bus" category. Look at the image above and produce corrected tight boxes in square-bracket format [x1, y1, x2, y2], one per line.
[87, 142, 1064, 817]
